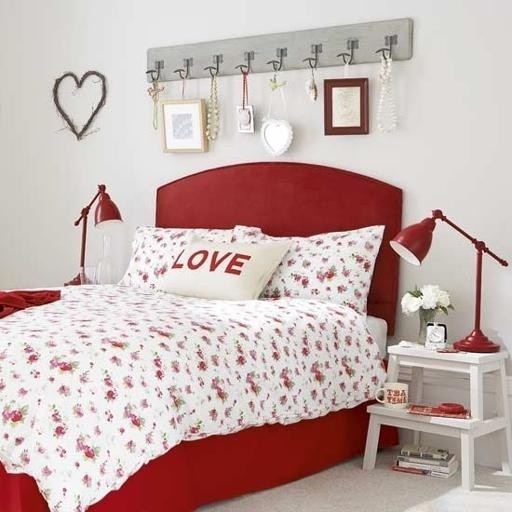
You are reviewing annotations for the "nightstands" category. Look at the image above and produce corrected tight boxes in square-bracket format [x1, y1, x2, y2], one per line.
[362, 342, 512, 489]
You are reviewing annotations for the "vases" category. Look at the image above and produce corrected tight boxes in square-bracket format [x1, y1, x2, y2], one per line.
[417, 309, 435, 345]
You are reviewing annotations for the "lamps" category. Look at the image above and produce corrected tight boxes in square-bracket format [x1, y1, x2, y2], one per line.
[389, 209, 508, 353]
[74, 184, 123, 285]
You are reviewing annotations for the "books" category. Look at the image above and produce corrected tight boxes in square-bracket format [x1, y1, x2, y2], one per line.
[392, 443, 459, 478]
[406, 403, 469, 419]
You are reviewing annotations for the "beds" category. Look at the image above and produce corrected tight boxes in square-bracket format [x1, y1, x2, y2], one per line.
[0, 161, 402, 512]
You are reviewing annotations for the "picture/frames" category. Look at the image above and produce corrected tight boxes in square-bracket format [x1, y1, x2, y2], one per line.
[160, 98, 208, 153]
[324, 78, 369, 135]
[236, 105, 254, 134]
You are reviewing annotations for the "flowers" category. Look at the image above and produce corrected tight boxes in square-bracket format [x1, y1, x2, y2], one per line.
[401, 285, 454, 336]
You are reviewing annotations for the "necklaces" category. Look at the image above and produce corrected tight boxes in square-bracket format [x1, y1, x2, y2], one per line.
[375, 55, 398, 134]
[205, 74, 222, 144]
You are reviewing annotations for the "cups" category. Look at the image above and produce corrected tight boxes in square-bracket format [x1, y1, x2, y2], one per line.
[374, 381, 408, 409]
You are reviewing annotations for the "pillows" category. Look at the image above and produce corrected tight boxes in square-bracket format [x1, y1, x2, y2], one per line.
[159, 234, 291, 299]
[117, 222, 261, 295]
[232, 224, 386, 317]
